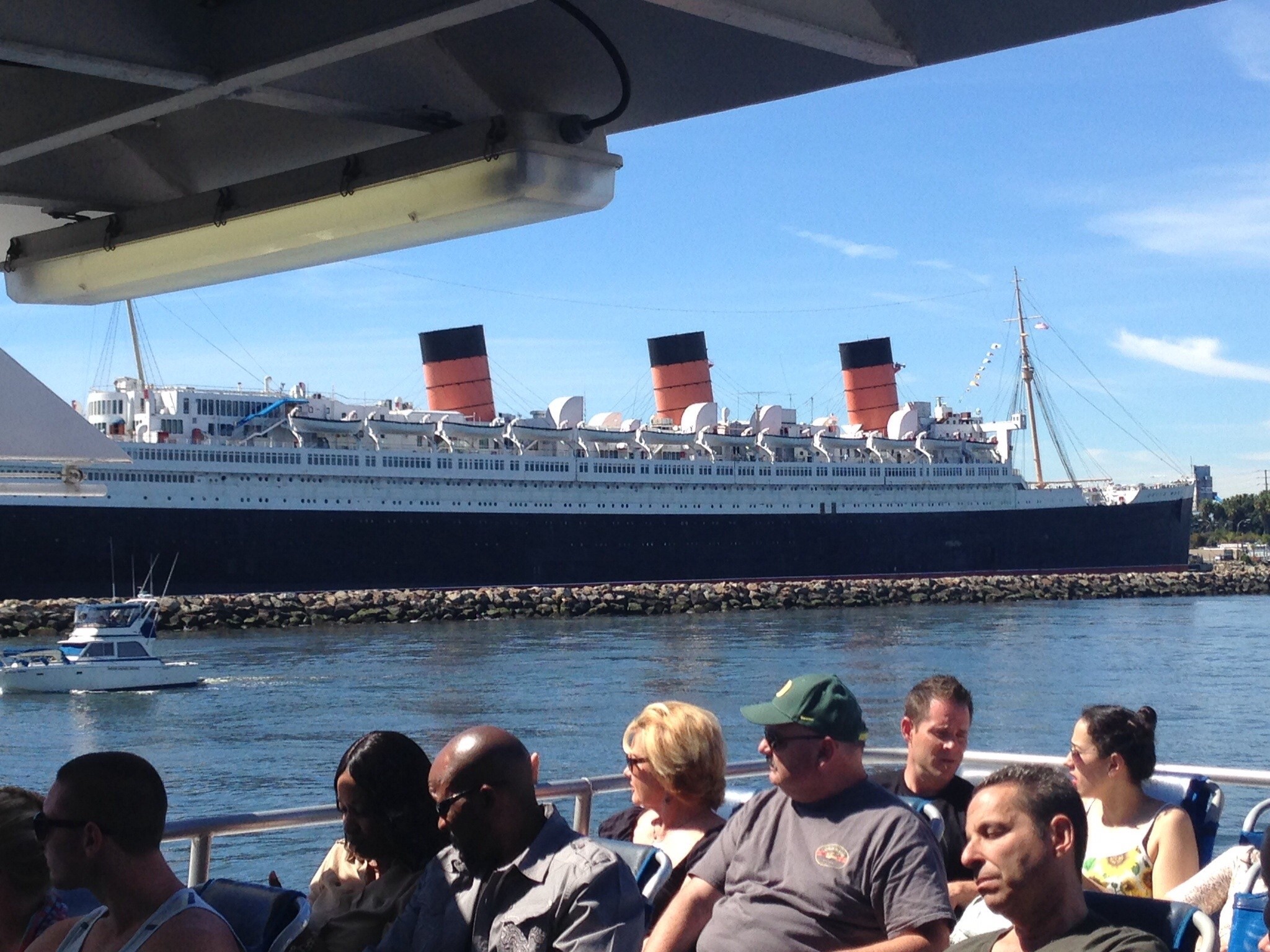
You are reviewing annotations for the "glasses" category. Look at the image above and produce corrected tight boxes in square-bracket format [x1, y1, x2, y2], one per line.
[626, 754, 650, 772]
[436, 780, 511, 818]
[33, 812, 114, 842]
[764, 727, 825, 749]
[1070, 748, 1111, 763]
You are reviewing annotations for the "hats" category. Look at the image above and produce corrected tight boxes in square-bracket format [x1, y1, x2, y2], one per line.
[740, 673, 868, 743]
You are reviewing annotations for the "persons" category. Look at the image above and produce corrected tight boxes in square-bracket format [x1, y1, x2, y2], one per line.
[0, 671, 1270, 952]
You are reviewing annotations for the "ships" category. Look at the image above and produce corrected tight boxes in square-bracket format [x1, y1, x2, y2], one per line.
[0, 267, 1216, 603]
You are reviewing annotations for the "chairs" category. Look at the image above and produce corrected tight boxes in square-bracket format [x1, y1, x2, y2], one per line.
[8, 774, 1270, 952]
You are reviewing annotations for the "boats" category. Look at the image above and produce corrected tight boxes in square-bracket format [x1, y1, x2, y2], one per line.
[0, 535, 205, 695]
